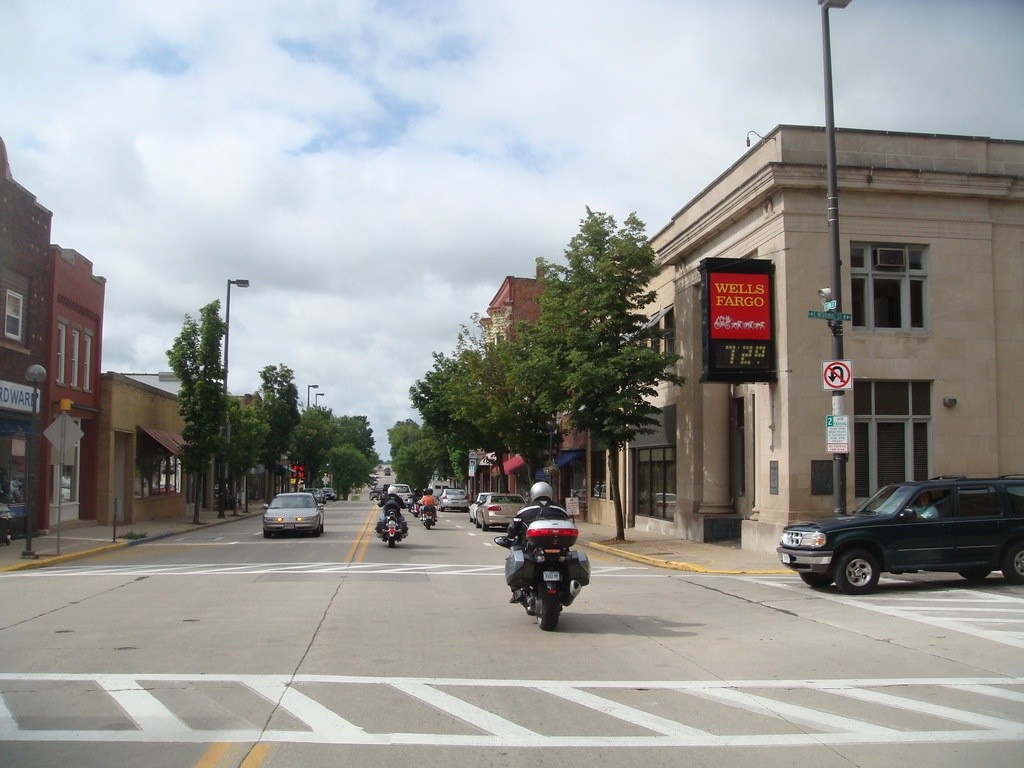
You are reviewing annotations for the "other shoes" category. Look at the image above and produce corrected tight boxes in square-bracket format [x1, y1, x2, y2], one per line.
[510, 589, 522, 603]
[402, 533, 408, 537]
[376, 532, 381, 538]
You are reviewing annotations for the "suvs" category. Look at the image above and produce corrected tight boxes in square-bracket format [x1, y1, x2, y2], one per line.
[775, 474, 1024, 594]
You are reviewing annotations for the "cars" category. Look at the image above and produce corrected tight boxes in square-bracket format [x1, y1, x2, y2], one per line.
[371, 464, 390, 484]
[468, 492, 527, 531]
[640, 492, 677, 520]
[8, 480, 23, 495]
[300, 488, 337, 504]
[151, 483, 175, 495]
[574, 485, 606, 501]
[369, 484, 391, 501]
[438, 489, 469, 512]
[263, 492, 325, 538]
[61, 476, 71, 501]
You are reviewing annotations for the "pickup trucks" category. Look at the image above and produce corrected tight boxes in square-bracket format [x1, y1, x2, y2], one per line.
[391, 484, 413, 505]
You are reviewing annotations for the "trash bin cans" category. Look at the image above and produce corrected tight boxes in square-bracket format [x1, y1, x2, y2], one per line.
[0, 503, 13, 545]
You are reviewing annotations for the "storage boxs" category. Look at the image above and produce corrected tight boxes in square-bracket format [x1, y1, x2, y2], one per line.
[526, 519, 579, 548]
[566, 550, 591, 586]
[400, 522, 408, 534]
[505, 549, 535, 588]
[376, 522, 385, 533]
[385, 500, 400, 511]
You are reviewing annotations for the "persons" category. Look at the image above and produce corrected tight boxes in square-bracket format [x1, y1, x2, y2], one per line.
[900, 492, 939, 520]
[510, 482, 569, 603]
[374, 485, 437, 539]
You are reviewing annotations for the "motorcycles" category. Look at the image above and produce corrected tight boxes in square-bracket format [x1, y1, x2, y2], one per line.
[494, 517, 591, 631]
[407, 494, 437, 529]
[377, 503, 409, 547]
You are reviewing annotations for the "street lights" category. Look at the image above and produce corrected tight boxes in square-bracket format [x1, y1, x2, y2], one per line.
[217, 278, 249, 518]
[306, 383, 324, 408]
[817, 0, 851, 515]
[544, 419, 557, 486]
[20, 365, 47, 560]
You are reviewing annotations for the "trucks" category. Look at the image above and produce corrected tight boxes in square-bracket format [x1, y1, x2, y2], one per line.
[432, 480, 450, 498]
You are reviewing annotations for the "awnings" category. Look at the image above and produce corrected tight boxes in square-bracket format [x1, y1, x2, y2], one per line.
[136, 426, 198, 457]
[491, 453, 580, 482]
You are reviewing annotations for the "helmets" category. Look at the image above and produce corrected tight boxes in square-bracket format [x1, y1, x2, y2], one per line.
[530, 481, 553, 502]
[414, 488, 418, 493]
[423, 488, 429, 494]
[387, 487, 398, 495]
